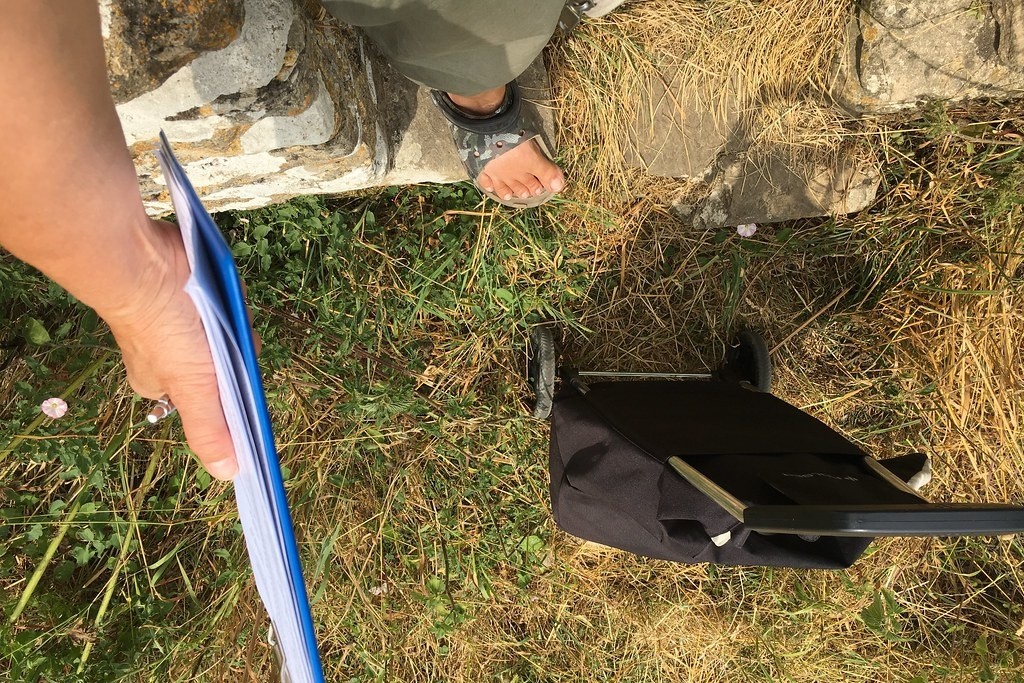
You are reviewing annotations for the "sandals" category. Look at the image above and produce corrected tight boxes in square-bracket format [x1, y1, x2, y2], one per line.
[430, 79, 566, 209]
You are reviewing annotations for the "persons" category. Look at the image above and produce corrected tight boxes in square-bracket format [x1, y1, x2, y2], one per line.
[0, 0, 569, 481]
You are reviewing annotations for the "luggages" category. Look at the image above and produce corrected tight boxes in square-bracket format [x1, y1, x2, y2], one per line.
[529, 327, 1024, 568]
